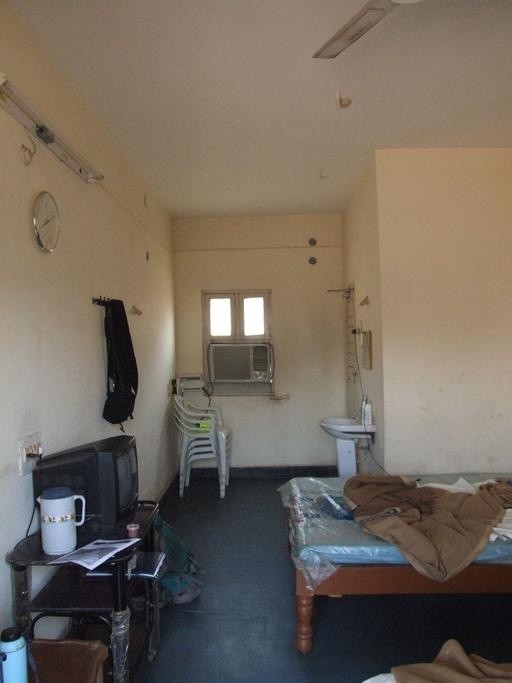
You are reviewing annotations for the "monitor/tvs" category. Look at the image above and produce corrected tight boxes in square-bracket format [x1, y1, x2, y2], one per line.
[33, 436, 138, 525]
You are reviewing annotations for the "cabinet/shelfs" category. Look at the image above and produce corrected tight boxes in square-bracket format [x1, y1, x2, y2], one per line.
[4, 500, 161, 683]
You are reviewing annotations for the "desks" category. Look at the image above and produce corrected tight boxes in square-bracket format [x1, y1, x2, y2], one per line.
[361, 671, 512, 683]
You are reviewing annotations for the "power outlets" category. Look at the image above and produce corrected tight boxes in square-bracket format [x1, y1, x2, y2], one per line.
[16, 429, 43, 477]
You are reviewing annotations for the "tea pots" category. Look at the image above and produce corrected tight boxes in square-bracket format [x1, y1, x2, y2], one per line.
[37, 487, 87, 556]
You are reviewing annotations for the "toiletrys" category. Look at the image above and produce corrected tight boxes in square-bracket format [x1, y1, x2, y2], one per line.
[361, 396, 372, 425]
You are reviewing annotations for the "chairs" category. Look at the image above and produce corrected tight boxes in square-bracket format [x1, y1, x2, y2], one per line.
[171, 395, 232, 500]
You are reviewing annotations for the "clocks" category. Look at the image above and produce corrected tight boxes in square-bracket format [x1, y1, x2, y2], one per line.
[33, 189, 60, 253]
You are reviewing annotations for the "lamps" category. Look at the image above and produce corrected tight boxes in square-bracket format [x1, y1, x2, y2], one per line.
[0, 72, 106, 186]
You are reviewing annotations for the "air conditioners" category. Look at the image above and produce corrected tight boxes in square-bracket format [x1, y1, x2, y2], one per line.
[207, 341, 273, 385]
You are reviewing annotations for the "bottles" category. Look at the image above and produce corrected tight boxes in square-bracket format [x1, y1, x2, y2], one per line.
[0, 628, 27, 683]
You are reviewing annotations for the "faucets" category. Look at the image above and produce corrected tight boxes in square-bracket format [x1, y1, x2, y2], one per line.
[349, 415, 360, 424]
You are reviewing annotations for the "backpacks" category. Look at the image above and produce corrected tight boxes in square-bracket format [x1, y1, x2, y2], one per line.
[153, 516, 202, 609]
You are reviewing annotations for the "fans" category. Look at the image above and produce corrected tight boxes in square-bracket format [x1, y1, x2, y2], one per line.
[311, 0, 429, 60]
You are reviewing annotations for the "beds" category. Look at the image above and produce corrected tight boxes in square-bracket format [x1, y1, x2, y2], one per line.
[290, 470, 512, 655]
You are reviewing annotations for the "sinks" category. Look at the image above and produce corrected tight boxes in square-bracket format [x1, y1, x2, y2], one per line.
[320, 417, 375, 440]
